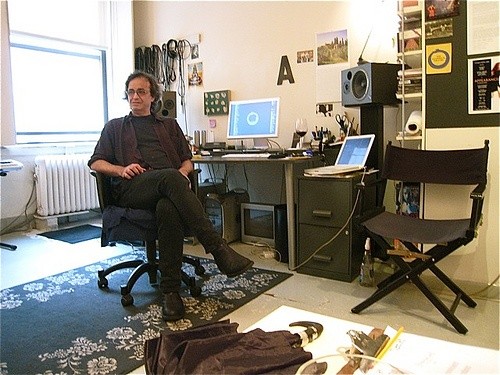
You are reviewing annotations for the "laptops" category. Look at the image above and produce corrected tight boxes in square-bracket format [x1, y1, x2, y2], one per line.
[305, 134, 375, 174]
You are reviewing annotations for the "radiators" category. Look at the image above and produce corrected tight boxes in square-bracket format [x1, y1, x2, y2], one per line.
[31, 151, 100, 216]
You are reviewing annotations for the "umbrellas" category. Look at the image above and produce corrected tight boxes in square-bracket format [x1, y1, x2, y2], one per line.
[145, 320, 327, 375]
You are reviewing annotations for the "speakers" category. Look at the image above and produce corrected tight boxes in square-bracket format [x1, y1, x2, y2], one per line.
[151, 91, 176, 118]
[341, 63, 402, 106]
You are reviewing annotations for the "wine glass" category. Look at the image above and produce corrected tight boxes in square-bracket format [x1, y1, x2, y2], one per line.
[294, 117, 308, 150]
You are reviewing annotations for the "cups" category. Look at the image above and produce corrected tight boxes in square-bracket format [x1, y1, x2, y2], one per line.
[295, 353, 409, 375]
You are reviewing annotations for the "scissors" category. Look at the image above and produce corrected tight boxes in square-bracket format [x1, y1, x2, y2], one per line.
[335, 114, 347, 128]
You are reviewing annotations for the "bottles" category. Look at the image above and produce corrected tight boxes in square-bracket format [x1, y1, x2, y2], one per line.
[359, 237, 375, 288]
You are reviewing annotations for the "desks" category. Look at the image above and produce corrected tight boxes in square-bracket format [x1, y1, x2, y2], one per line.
[241, 304, 499, 375]
[186, 154, 316, 271]
[0, 160, 24, 250]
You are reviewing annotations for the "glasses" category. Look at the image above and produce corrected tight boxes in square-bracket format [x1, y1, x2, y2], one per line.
[126, 89, 151, 96]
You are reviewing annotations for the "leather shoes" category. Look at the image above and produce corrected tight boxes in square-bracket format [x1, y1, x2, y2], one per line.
[163, 293, 186, 321]
[211, 242, 254, 277]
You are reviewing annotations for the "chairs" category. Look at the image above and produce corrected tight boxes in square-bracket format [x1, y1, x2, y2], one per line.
[350, 139, 490, 334]
[91, 170, 209, 306]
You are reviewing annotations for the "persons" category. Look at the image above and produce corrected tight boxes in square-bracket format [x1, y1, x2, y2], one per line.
[88, 72, 253, 321]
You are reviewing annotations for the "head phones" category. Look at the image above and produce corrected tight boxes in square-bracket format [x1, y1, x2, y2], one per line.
[167, 39, 177, 58]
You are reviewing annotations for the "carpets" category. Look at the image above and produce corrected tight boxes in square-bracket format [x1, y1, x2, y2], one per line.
[36, 224, 103, 245]
[1, 247, 294, 375]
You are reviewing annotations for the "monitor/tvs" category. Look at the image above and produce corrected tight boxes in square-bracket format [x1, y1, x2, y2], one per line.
[227, 97, 280, 147]
[240, 202, 276, 247]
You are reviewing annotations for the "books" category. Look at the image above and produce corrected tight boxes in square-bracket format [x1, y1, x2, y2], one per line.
[396, 68, 422, 93]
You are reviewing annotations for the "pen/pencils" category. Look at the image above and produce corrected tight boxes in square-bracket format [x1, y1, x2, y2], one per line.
[312, 125, 331, 142]
[368, 326, 404, 370]
[345, 111, 359, 136]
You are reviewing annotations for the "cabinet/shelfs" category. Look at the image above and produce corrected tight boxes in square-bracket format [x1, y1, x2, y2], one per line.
[396, 0, 426, 263]
[294, 169, 380, 284]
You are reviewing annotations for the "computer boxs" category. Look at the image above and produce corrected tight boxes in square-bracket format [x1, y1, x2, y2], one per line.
[202, 188, 249, 244]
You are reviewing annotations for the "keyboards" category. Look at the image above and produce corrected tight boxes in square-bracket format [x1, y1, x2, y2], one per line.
[222, 154, 271, 158]
